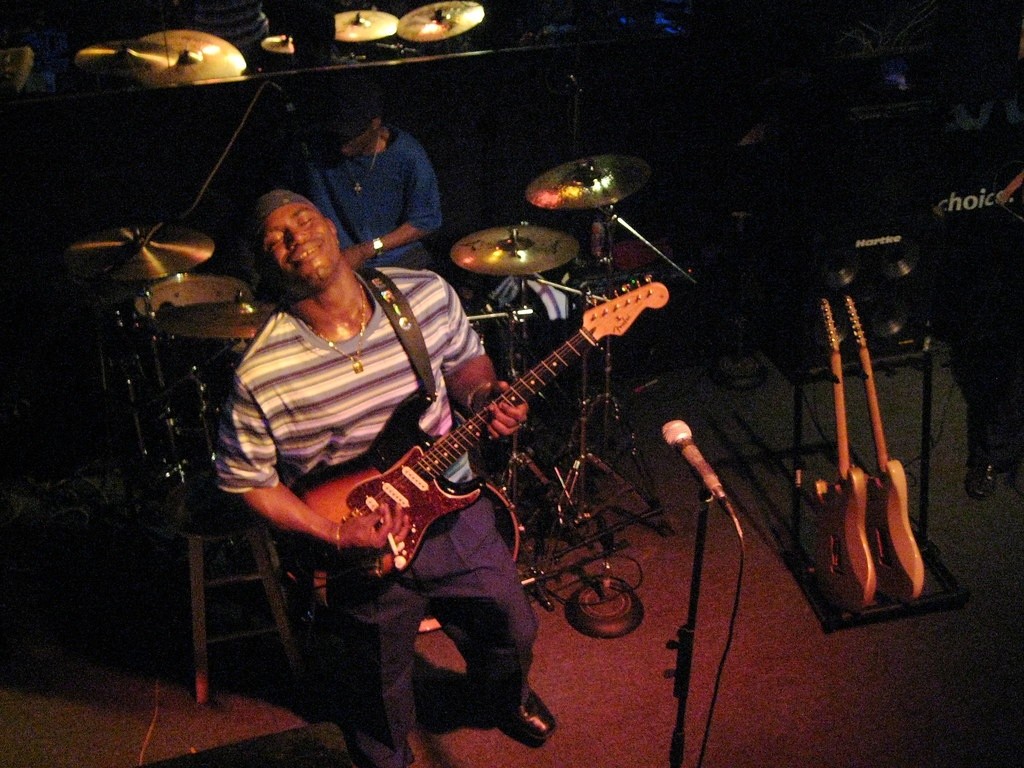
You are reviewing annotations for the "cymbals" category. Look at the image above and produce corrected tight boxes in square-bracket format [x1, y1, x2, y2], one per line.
[524, 154, 652, 210]
[397, 0, 487, 43]
[134, 301, 277, 341]
[333, 9, 399, 43]
[75, 39, 128, 79]
[121, 29, 248, 88]
[66, 225, 217, 281]
[451, 224, 581, 275]
[261, 33, 294, 55]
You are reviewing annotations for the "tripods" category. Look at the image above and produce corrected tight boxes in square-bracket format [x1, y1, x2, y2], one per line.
[498, 204, 678, 588]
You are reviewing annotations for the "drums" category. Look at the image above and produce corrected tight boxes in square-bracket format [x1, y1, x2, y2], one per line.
[134, 272, 253, 321]
[306, 479, 521, 632]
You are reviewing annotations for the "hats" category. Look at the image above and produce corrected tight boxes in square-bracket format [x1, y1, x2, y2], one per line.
[248, 189, 320, 233]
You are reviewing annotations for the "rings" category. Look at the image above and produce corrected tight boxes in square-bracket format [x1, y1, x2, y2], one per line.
[519, 421, 524, 427]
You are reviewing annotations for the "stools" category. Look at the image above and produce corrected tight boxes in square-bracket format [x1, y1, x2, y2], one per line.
[164, 472, 306, 704]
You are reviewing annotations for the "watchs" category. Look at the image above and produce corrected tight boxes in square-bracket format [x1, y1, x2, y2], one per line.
[372, 236, 382, 258]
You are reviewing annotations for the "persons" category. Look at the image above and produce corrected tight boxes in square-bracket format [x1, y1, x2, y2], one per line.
[294, 86, 443, 275]
[212, 188, 556, 767]
[931, 0, 1024, 500]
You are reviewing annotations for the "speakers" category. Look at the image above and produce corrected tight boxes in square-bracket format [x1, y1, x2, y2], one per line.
[762, 69, 940, 383]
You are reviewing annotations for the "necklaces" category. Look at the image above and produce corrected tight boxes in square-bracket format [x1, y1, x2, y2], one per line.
[342, 133, 381, 197]
[281, 276, 367, 374]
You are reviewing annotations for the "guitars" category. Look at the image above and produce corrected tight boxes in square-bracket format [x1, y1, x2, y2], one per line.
[839, 296, 925, 604]
[292, 273, 670, 580]
[814, 297, 877, 612]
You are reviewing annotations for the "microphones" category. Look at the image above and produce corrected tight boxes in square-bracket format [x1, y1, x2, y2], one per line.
[280, 88, 312, 161]
[662, 420, 736, 517]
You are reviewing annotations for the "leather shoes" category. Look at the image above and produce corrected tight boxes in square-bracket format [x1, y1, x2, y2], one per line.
[499, 688, 556, 742]
[965, 463, 996, 497]
[1012, 462, 1024, 496]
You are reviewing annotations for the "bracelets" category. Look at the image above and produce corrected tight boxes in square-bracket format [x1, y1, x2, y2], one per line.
[335, 525, 341, 550]
